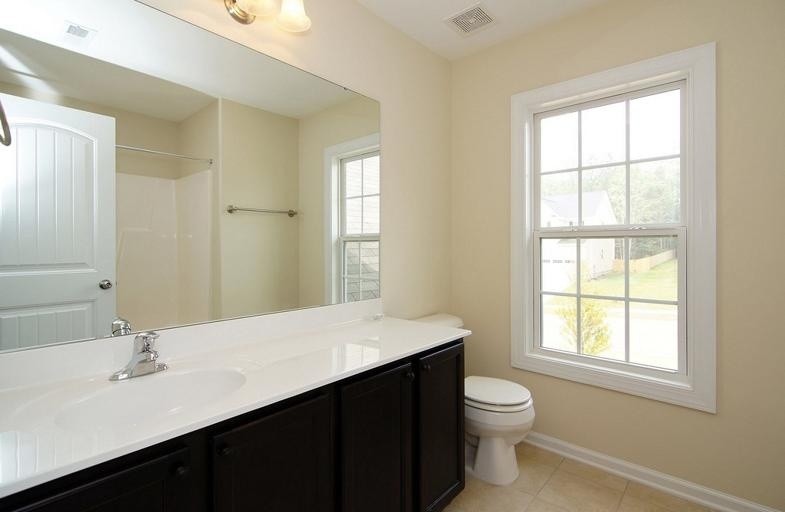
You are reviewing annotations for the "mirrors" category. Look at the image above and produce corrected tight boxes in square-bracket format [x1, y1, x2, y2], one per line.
[0, 0, 381, 353]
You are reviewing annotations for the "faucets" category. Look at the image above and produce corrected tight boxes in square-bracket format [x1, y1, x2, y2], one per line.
[111, 316, 133, 337]
[108, 330, 169, 381]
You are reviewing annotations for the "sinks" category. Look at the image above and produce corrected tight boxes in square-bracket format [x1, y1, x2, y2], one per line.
[10, 355, 266, 437]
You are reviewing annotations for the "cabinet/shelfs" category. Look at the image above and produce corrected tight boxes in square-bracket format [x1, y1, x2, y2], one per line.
[337, 338, 465, 512]
[0, 338, 337, 512]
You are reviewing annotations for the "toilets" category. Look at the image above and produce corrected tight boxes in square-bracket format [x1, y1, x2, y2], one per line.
[411, 311, 535, 488]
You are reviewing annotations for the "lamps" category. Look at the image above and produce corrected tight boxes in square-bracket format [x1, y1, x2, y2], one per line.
[224, 0, 311, 32]
